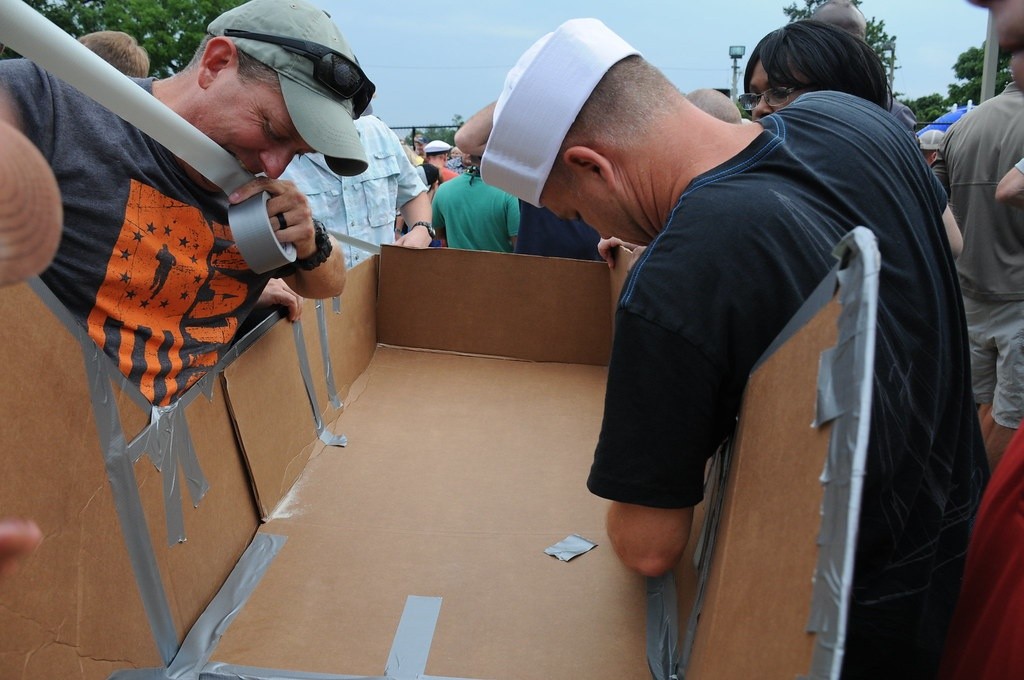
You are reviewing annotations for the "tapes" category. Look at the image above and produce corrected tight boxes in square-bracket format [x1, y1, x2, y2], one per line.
[0, 0, 297, 272]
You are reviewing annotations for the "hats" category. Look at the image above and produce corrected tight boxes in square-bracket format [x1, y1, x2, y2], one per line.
[480, 17, 641, 209]
[424, 139, 453, 153]
[207, 0, 369, 177]
[917, 129, 945, 150]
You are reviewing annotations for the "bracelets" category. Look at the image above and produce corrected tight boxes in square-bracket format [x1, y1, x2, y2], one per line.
[296, 219, 332, 271]
[395, 228, 402, 232]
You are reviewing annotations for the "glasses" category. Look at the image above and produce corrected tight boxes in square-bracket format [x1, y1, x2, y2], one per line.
[224, 29, 375, 120]
[738, 85, 814, 110]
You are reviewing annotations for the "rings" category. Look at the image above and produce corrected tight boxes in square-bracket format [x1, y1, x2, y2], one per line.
[276, 212, 287, 230]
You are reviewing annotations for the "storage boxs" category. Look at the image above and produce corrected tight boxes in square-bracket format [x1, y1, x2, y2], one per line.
[0, 224, 882, 680]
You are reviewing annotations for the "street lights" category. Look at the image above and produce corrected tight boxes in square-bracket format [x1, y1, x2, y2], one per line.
[728, 46, 745, 106]
[879, 42, 895, 97]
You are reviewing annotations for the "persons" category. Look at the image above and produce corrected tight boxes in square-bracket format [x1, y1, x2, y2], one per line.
[0, 0, 431, 565]
[424, 140, 603, 259]
[482, 17, 992, 680]
[918, 0, 1024, 470]
[688, 1, 917, 133]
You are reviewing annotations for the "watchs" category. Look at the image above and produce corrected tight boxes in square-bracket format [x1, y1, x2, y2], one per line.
[412, 222, 436, 238]
